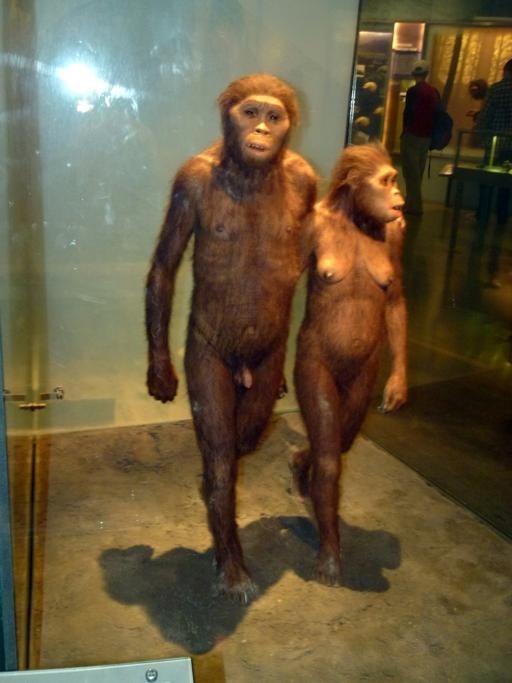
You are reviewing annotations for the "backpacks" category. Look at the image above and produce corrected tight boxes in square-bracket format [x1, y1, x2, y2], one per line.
[427, 111, 453, 181]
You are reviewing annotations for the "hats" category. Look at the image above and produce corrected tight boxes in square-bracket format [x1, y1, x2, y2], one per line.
[412, 59, 429, 74]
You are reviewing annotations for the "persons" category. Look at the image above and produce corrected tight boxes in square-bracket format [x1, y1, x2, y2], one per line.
[387, 58, 443, 217]
[465, 58, 512, 225]
